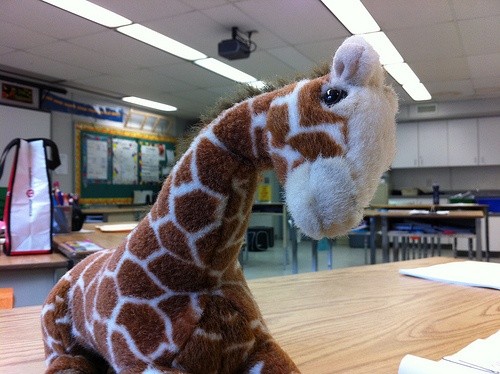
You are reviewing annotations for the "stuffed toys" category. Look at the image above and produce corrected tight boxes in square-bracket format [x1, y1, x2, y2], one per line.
[38, 35, 398, 374]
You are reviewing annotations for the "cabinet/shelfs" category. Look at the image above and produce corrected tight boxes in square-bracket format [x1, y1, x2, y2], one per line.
[391, 116, 500, 168]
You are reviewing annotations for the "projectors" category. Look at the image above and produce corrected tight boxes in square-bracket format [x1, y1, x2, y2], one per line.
[218, 39, 250, 59]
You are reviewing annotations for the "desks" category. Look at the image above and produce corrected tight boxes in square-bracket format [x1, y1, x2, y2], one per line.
[0, 204, 500, 374]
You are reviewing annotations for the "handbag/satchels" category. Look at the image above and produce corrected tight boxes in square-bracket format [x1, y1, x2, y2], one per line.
[0, 137, 60, 256]
